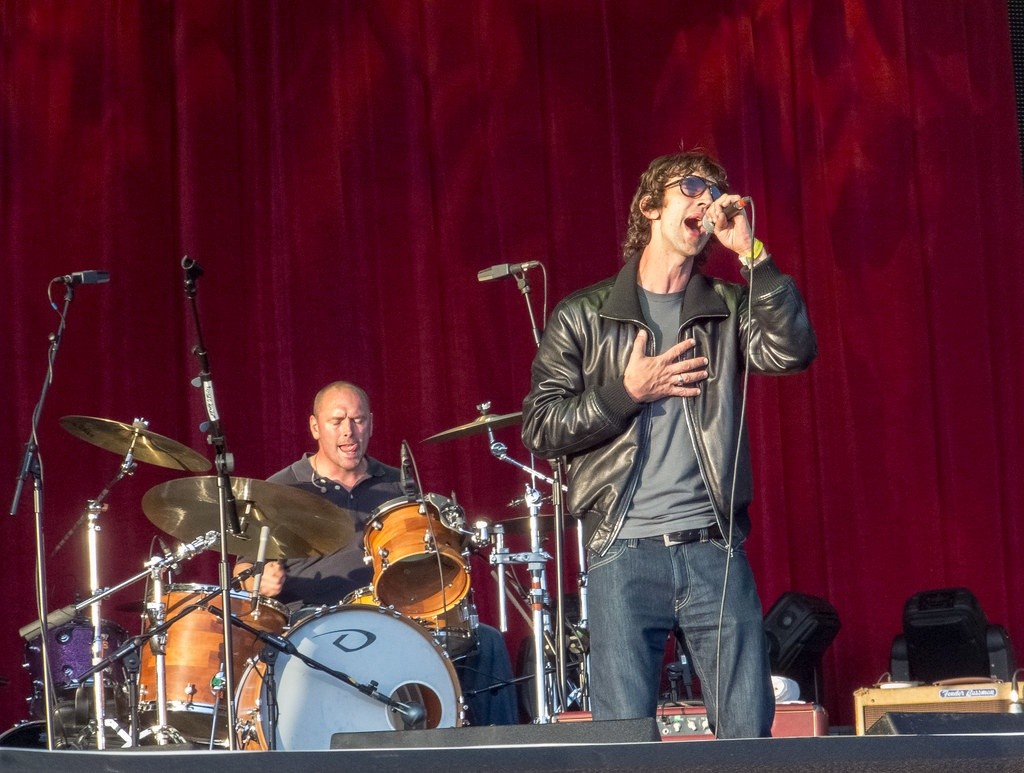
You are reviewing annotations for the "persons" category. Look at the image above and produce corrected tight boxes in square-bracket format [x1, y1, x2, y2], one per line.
[521, 151, 819, 740]
[233, 381, 518, 728]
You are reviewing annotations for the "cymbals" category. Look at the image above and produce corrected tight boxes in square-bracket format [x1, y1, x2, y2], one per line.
[141, 475, 354, 560]
[58, 415, 212, 472]
[492, 513, 576, 535]
[419, 411, 523, 446]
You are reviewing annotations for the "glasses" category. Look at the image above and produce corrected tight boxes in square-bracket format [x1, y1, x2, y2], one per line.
[664, 173, 727, 204]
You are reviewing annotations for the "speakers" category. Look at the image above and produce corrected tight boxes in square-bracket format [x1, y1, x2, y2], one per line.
[329, 717, 662, 750]
[855, 681, 1024, 735]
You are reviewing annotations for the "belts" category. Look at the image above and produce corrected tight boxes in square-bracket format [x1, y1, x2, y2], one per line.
[645, 525, 724, 547]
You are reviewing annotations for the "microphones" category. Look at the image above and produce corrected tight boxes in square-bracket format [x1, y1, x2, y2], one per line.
[703, 196, 753, 233]
[400, 443, 417, 495]
[54, 270, 110, 284]
[250, 525, 270, 610]
[1009, 678, 1024, 713]
[477, 261, 539, 283]
[395, 701, 426, 727]
[159, 538, 183, 575]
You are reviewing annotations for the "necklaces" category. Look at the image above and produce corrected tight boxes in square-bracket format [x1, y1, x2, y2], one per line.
[315, 455, 318, 469]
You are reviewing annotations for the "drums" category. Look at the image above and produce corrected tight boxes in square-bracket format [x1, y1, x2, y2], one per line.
[362, 493, 471, 620]
[232, 602, 466, 751]
[20, 618, 138, 720]
[343, 585, 479, 663]
[136, 583, 291, 746]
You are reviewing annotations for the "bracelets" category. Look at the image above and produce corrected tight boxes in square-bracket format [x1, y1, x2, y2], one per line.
[739, 240, 763, 265]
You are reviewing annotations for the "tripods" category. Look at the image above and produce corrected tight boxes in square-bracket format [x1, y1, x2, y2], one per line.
[17, 528, 223, 750]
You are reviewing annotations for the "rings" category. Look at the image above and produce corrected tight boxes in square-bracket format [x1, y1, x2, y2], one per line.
[678, 376, 684, 386]
